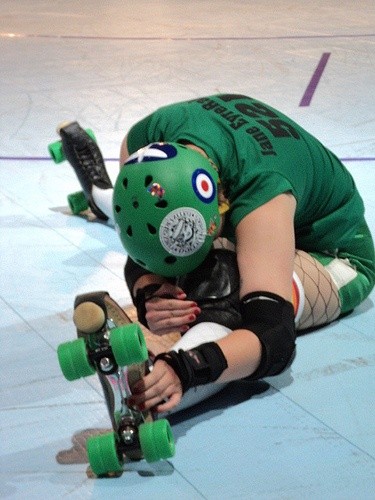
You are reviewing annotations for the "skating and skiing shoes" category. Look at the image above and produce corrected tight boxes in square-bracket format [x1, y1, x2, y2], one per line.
[47, 120, 117, 222]
[55, 286, 179, 477]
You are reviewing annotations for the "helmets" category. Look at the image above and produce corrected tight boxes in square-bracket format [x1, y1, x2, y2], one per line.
[112, 141, 218, 277]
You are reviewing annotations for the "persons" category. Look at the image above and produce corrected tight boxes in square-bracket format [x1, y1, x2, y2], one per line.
[59, 93, 375, 423]
